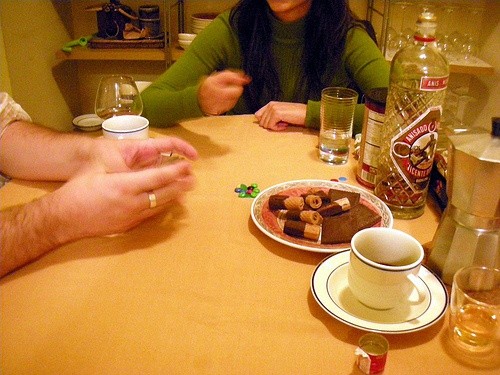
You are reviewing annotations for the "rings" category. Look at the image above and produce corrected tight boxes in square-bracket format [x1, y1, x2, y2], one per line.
[147, 190, 157, 209]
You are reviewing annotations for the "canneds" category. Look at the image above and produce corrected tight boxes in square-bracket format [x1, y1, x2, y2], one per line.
[356, 86, 387, 189]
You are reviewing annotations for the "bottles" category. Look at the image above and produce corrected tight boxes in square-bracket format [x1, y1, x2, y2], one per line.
[374, 13, 449, 219]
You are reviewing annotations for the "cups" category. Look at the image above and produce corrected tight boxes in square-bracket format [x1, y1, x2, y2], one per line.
[139, 5, 160, 37]
[102, 115, 149, 140]
[356, 87, 416, 192]
[449, 265, 500, 353]
[348, 227, 425, 310]
[94, 74, 144, 120]
[319, 87, 359, 166]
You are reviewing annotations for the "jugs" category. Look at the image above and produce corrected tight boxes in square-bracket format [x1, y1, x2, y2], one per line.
[426, 117, 500, 292]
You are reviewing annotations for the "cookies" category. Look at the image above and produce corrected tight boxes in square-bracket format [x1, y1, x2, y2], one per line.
[269, 189, 383, 244]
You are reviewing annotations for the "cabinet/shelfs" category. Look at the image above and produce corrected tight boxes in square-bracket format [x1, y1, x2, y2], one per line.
[55, 0, 494, 113]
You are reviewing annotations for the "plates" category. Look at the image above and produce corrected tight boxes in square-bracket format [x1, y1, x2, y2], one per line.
[251, 180, 393, 253]
[310, 249, 450, 334]
[190, 12, 220, 34]
[72, 113, 104, 131]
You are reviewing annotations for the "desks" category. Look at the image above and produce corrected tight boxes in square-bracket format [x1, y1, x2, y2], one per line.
[0, 113, 500, 375]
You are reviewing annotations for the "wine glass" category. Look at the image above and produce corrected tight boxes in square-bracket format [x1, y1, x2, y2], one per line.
[381, 2, 484, 65]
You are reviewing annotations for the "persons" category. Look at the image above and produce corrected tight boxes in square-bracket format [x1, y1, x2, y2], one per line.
[132, 0, 392, 137]
[0, 92, 199, 282]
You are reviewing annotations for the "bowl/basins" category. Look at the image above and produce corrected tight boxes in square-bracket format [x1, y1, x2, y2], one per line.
[178, 39, 192, 51]
[178, 34, 196, 41]
